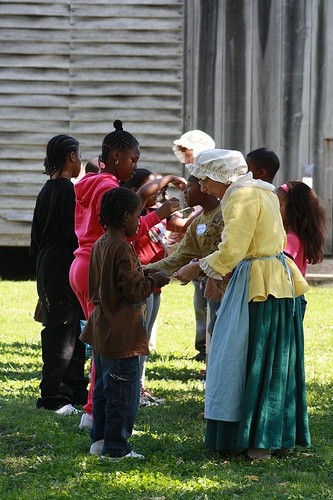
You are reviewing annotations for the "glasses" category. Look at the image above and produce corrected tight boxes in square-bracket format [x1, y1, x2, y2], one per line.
[198, 177, 209, 186]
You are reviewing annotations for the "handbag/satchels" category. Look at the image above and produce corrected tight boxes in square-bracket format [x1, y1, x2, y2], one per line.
[33, 283, 80, 326]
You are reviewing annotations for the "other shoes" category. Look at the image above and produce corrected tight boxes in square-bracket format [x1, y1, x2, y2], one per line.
[220, 448, 272, 463]
[55, 404, 78, 416]
[78, 413, 92, 430]
[90, 439, 104, 456]
[138, 386, 166, 408]
[101, 450, 146, 461]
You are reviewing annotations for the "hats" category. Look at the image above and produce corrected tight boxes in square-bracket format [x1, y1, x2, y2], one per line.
[184, 148, 248, 185]
[172, 130, 215, 163]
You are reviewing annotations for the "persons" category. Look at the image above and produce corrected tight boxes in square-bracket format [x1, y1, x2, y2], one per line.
[29, 120, 325, 458]
